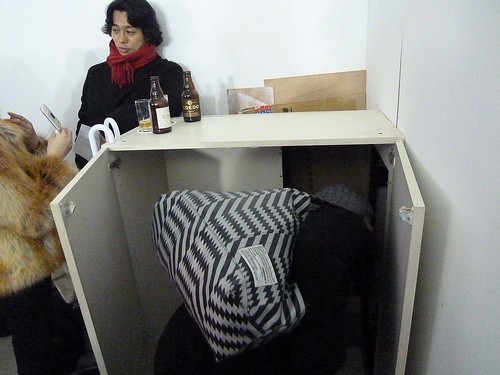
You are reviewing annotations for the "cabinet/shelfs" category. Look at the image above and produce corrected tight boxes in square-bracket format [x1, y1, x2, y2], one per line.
[49, 108, 426, 375]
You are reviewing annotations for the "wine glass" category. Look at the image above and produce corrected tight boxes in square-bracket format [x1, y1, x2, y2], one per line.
[133, 98, 153, 133]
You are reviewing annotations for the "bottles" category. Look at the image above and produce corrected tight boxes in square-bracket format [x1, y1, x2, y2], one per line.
[180, 71, 201, 122]
[148, 76, 172, 133]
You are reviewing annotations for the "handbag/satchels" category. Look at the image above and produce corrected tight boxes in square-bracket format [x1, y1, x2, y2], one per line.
[150, 187, 323, 362]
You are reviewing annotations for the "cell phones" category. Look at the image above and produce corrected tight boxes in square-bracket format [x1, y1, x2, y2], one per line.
[39, 104, 61, 142]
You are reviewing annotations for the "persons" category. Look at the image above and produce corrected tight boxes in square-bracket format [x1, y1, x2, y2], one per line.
[75, 0, 183, 171]
[0, 111, 100, 375]
